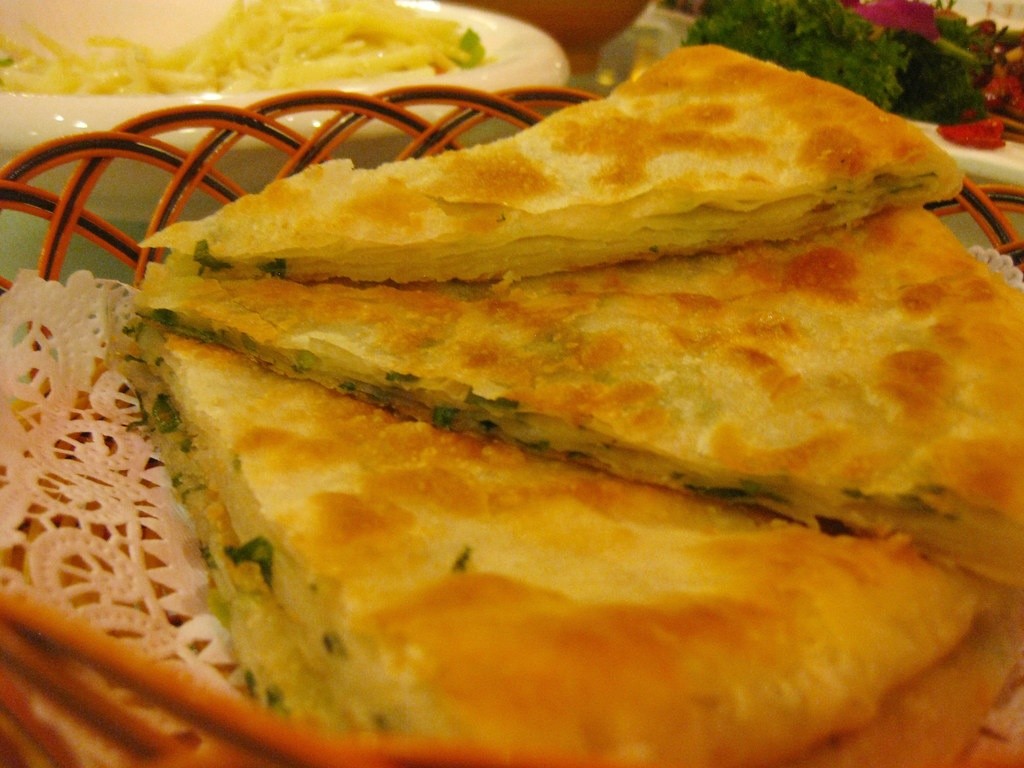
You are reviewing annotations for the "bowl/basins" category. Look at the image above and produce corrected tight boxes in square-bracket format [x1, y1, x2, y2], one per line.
[0, 0, 570, 223]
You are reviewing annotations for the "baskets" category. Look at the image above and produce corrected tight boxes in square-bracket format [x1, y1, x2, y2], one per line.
[0, 86, 1023, 767]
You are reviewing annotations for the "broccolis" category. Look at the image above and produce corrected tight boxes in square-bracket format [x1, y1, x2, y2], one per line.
[682, 0, 986, 124]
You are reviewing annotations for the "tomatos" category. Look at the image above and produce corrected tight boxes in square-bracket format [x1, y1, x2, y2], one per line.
[938, 120, 1005, 149]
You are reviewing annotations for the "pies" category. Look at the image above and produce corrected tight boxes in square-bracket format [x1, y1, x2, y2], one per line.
[139, 40, 1024, 767]
